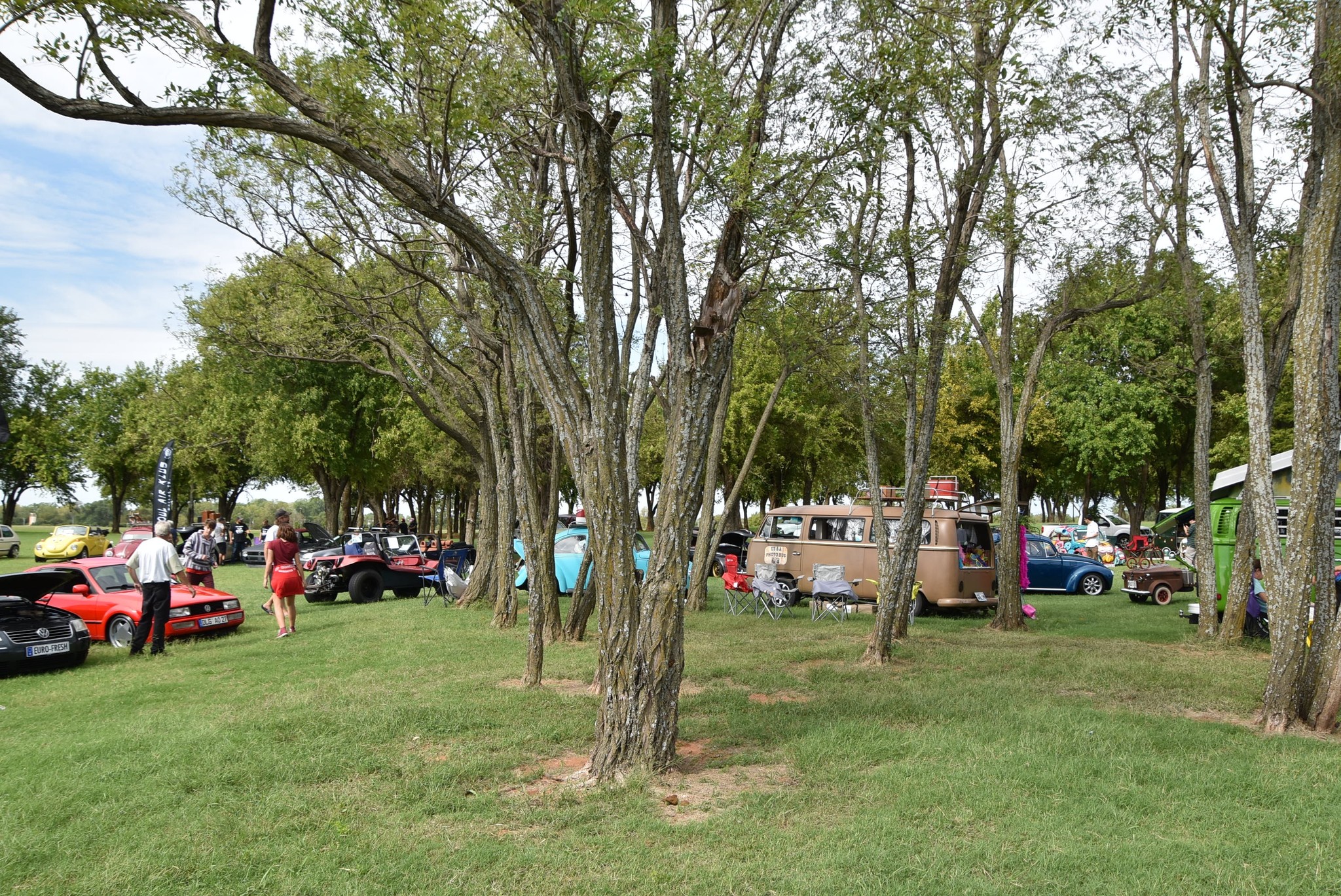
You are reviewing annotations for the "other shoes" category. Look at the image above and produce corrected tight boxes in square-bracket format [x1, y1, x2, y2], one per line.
[129, 648, 167, 656]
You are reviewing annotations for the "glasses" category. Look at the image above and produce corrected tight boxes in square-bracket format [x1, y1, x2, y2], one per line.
[1084, 519, 1087, 522]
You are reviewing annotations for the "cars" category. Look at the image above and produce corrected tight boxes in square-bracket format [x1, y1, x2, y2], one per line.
[176, 525, 244, 564]
[103, 521, 167, 559]
[23, 557, 245, 649]
[0, 525, 21, 559]
[34, 525, 114, 563]
[241, 529, 312, 564]
[990, 524, 1114, 596]
[1047, 525, 1126, 565]
[513, 520, 696, 598]
[0, 571, 98, 679]
[398, 534, 477, 573]
[687, 526, 759, 579]
[292, 522, 405, 571]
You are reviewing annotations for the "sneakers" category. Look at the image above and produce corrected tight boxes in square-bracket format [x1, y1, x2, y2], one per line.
[289, 626, 296, 633]
[275, 627, 289, 638]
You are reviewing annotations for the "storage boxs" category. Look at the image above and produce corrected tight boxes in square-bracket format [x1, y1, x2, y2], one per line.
[201, 510, 215, 523]
[925, 475, 959, 497]
[209, 513, 221, 522]
[878, 485, 896, 498]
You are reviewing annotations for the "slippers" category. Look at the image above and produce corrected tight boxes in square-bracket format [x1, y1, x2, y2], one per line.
[261, 605, 273, 615]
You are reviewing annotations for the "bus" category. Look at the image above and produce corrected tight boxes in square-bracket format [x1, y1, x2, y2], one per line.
[746, 476, 1000, 617]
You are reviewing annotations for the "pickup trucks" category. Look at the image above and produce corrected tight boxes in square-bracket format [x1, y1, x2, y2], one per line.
[1041, 514, 1151, 546]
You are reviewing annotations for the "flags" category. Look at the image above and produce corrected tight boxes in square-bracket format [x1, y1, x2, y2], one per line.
[153, 438, 176, 524]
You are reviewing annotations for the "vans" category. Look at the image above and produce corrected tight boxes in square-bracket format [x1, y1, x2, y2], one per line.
[1121, 496, 1341, 637]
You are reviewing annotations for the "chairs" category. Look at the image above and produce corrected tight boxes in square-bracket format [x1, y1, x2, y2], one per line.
[440, 540, 446, 550]
[721, 554, 758, 616]
[1241, 574, 1270, 642]
[419, 541, 429, 552]
[865, 573, 923, 627]
[426, 541, 437, 552]
[445, 540, 453, 549]
[750, 563, 805, 622]
[418, 548, 470, 608]
[808, 563, 863, 623]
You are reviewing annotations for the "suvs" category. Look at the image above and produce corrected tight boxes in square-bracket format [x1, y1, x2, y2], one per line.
[303, 531, 439, 605]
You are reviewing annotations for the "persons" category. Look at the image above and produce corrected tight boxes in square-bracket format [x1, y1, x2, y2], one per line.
[399, 518, 408, 535]
[214, 517, 226, 566]
[1182, 516, 1197, 587]
[229, 516, 251, 565]
[243, 531, 252, 548]
[259, 519, 272, 543]
[1082, 514, 1099, 561]
[370, 523, 381, 532]
[427, 536, 434, 542]
[181, 518, 219, 589]
[382, 517, 395, 533]
[263, 522, 307, 640]
[125, 520, 196, 655]
[176, 532, 184, 554]
[165, 532, 173, 544]
[408, 518, 418, 535]
[260, 509, 293, 616]
[1244, 559, 1268, 613]
[166, 520, 179, 548]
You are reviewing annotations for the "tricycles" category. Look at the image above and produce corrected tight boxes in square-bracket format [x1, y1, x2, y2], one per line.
[1126, 536, 1165, 570]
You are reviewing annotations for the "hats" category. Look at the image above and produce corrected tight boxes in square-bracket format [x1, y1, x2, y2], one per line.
[401, 518, 405, 522]
[237, 516, 244, 520]
[409, 518, 415, 522]
[218, 517, 226, 522]
[275, 509, 291, 520]
